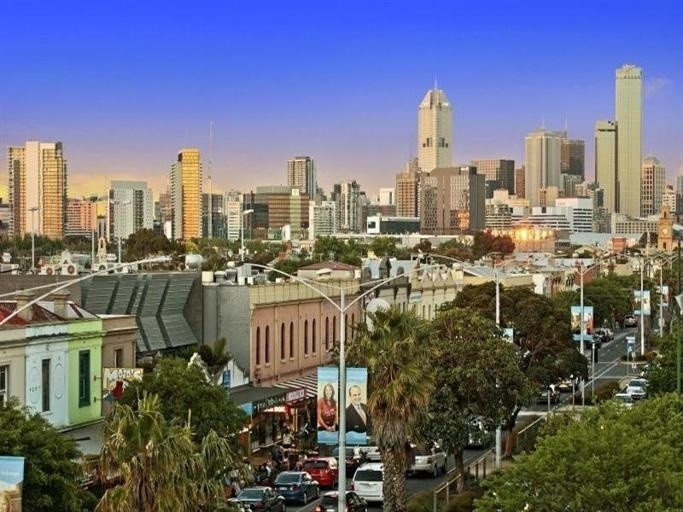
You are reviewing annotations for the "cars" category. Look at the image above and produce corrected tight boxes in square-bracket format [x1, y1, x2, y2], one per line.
[613, 364, 650, 409]
[587, 327, 614, 348]
[536, 375, 578, 403]
[407, 439, 448, 478]
[466, 416, 508, 450]
[228, 445, 385, 512]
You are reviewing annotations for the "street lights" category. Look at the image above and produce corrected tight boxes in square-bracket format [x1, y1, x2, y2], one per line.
[527, 242, 683, 393]
[241, 208, 255, 264]
[27, 206, 39, 276]
[417, 252, 553, 474]
[109, 199, 130, 264]
[226, 260, 462, 511]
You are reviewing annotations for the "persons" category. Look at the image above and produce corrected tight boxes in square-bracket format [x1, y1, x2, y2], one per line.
[261, 463, 271, 477]
[315, 383, 339, 432]
[313, 502, 324, 512]
[344, 385, 369, 434]
[273, 429, 297, 470]
